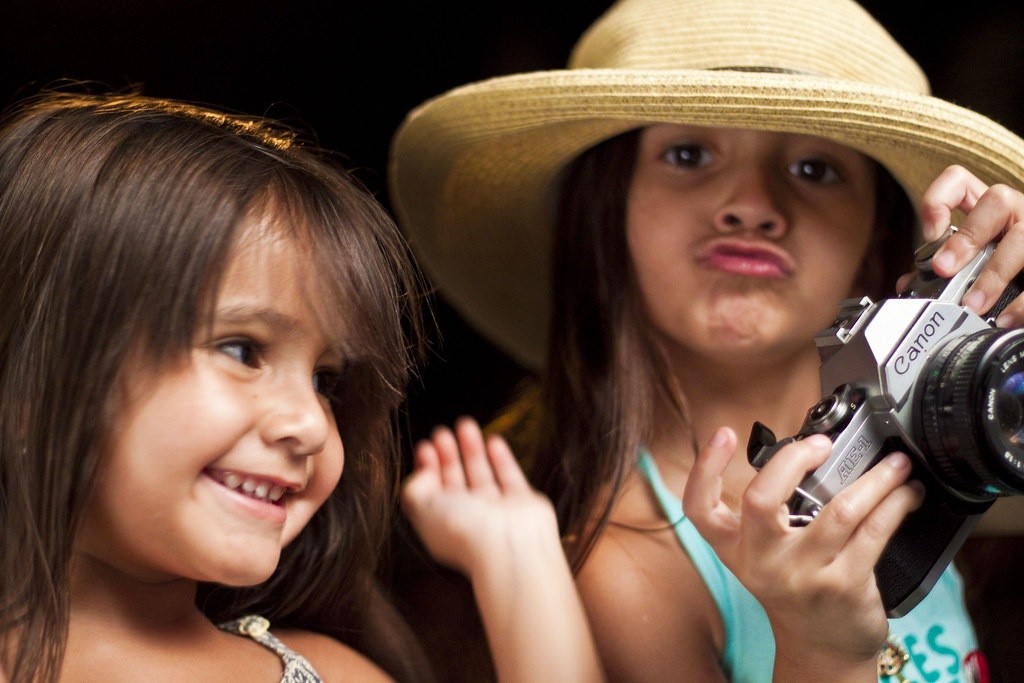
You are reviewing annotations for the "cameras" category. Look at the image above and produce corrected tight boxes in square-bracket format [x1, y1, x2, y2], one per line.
[746, 226, 1024, 619]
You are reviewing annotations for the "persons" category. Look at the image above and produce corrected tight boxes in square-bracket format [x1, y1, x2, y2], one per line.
[526, 121, 1024, 683]
[0, 87, 610, 683]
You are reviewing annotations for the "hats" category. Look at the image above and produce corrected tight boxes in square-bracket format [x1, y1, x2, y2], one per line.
[387, 0, 1024, 372]
